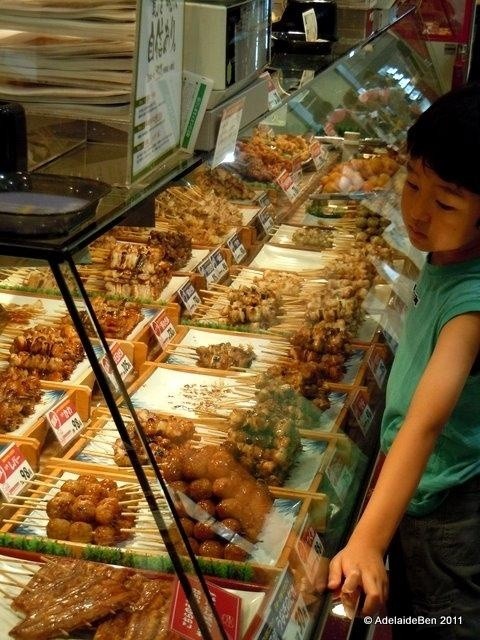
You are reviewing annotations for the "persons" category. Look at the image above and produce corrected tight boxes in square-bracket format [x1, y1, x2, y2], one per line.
[264, 517, 332, 626]
[323, 85, 480, 639]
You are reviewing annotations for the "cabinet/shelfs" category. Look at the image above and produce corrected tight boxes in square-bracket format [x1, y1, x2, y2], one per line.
[0, 0, 478, 640]
